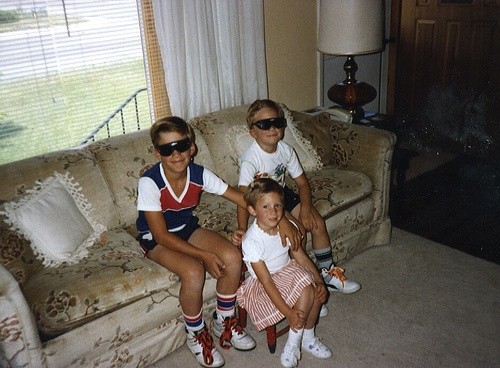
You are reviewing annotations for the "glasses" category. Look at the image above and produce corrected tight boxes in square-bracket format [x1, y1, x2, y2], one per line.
[252, 117, 287, 130]
[155, 137, 191, 157]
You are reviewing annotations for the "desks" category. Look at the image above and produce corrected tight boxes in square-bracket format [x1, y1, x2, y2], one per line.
[354, 111, 392, 131]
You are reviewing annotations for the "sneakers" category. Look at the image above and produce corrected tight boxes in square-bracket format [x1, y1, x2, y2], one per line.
[301, 337, 332, 359]
[185, 321, 224, 368]
[280, 339, 301, 368]
[319, 304, 328, 317]
[210, 306, 257, 351]
[320, 263, 360, 294]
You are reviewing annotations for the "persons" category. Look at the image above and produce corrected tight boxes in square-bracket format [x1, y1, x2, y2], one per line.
[236, 178, 333, 368]
[237, 99, 361, 318]
[135, 116, 303, 368]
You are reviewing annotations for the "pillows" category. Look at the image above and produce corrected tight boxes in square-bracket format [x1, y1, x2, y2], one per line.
[227, 102, 322, 176]
[0, 170, 110, 270]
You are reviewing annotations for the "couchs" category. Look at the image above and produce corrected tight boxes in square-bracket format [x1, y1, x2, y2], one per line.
[0, 101, 398, 367]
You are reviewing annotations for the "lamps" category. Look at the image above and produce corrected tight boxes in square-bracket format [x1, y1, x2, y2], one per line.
[318, 0, 385, 121]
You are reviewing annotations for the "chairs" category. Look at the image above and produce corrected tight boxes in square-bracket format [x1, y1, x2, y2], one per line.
[238, 249, 290, 355]
